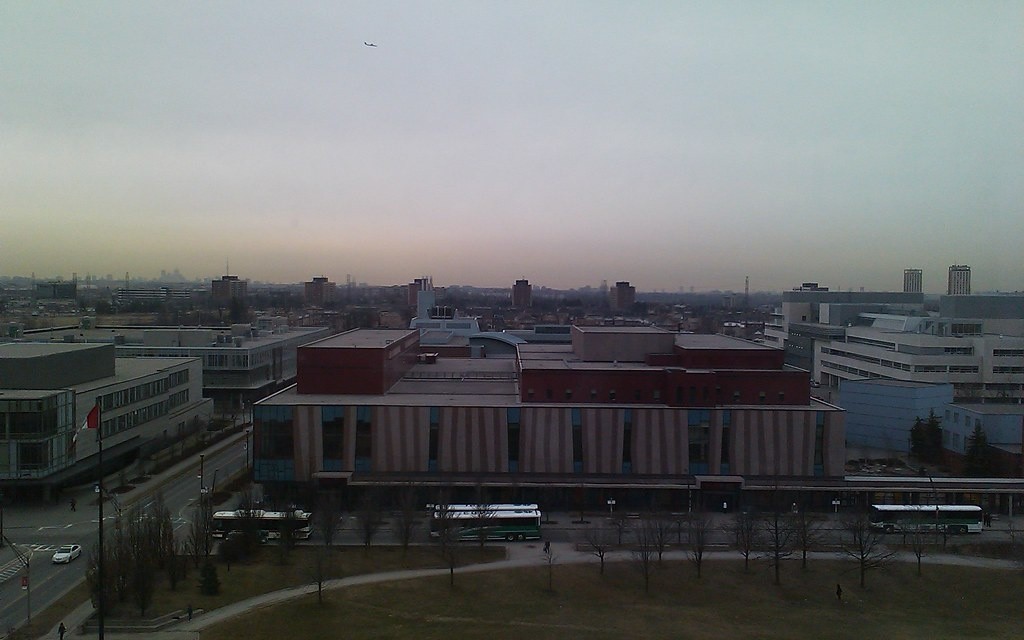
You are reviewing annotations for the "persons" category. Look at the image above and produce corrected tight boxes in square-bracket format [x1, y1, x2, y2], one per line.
[984, 512, 992, 527]
[71, 503, 76, 512]
[58, 623, 66, 640]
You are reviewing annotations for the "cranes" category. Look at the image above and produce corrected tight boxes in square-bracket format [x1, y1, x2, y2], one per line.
[125, 272, 129, 289]
[745, 276, 749, 296]
[346, 274, 351, 285]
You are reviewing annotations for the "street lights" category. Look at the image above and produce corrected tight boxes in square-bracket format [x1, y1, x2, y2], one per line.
[200, 454, 204, 501]
[246, 429, 249, 470]
[212, 469, 218, 491]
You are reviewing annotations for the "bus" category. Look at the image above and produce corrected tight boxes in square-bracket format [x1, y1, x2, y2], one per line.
[210, 509, 314, 539]
[869, 504, 983, 535]
[430, 504, 542, 542]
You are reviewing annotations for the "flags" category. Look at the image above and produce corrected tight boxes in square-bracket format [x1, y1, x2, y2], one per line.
[72, 402, 98, 443]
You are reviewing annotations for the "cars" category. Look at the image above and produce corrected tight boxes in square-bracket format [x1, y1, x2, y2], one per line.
[32, 311, 39, 316]
[810, 380, 820, 388]
[52, 544, 81, 564]
